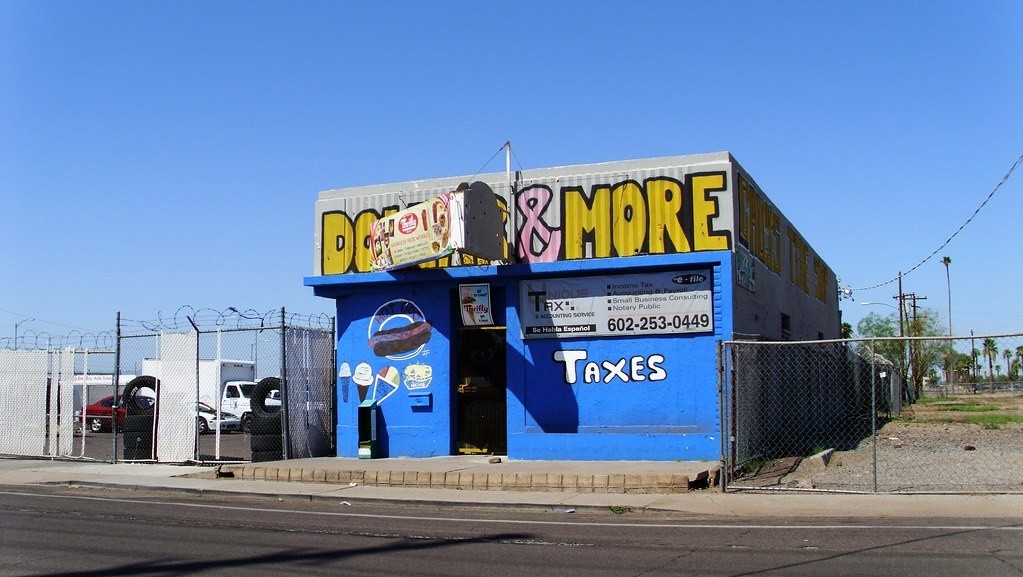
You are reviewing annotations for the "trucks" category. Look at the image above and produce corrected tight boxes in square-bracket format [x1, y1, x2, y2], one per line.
[140, 357, 282, 435]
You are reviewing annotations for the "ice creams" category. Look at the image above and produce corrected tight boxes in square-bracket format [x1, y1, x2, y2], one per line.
[462, 287, 476, 324]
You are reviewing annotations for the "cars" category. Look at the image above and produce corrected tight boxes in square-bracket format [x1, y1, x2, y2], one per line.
[197, 401, 242, 435]
[79, 394, 155, 434]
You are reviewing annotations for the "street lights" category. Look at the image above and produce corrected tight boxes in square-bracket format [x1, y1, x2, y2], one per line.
[940, 256, 954, 395]
[862, 302, 903, 374]
